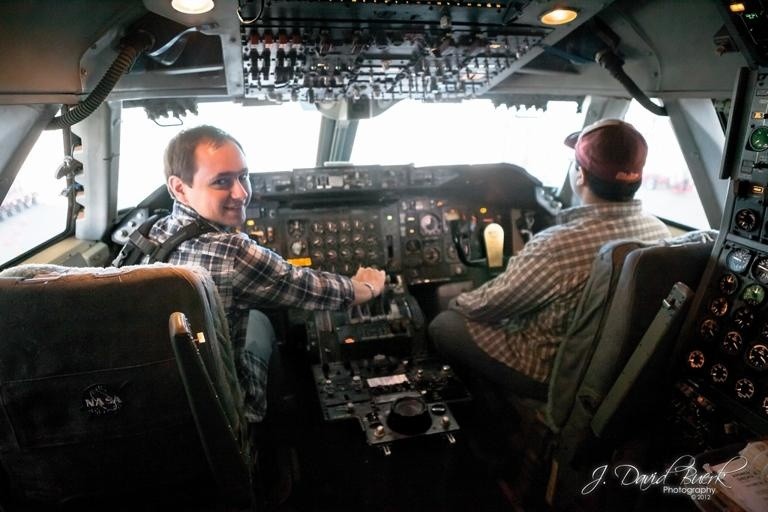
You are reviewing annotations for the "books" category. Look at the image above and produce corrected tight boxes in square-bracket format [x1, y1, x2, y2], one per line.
[683, 438, 767, 511]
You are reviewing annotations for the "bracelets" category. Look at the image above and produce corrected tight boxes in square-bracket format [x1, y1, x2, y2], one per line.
[362, 282, 376, 301]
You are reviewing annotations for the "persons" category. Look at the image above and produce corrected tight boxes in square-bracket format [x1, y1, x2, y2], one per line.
[105, 124, 392, 433]
[430, 118, 675, 447]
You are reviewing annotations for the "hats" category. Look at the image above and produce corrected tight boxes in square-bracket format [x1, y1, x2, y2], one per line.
[563, 118, 648, 183]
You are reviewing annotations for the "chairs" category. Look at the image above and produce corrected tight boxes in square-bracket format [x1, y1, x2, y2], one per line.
[507, 229, 719, 465]
[0, 259, 255, 511]
